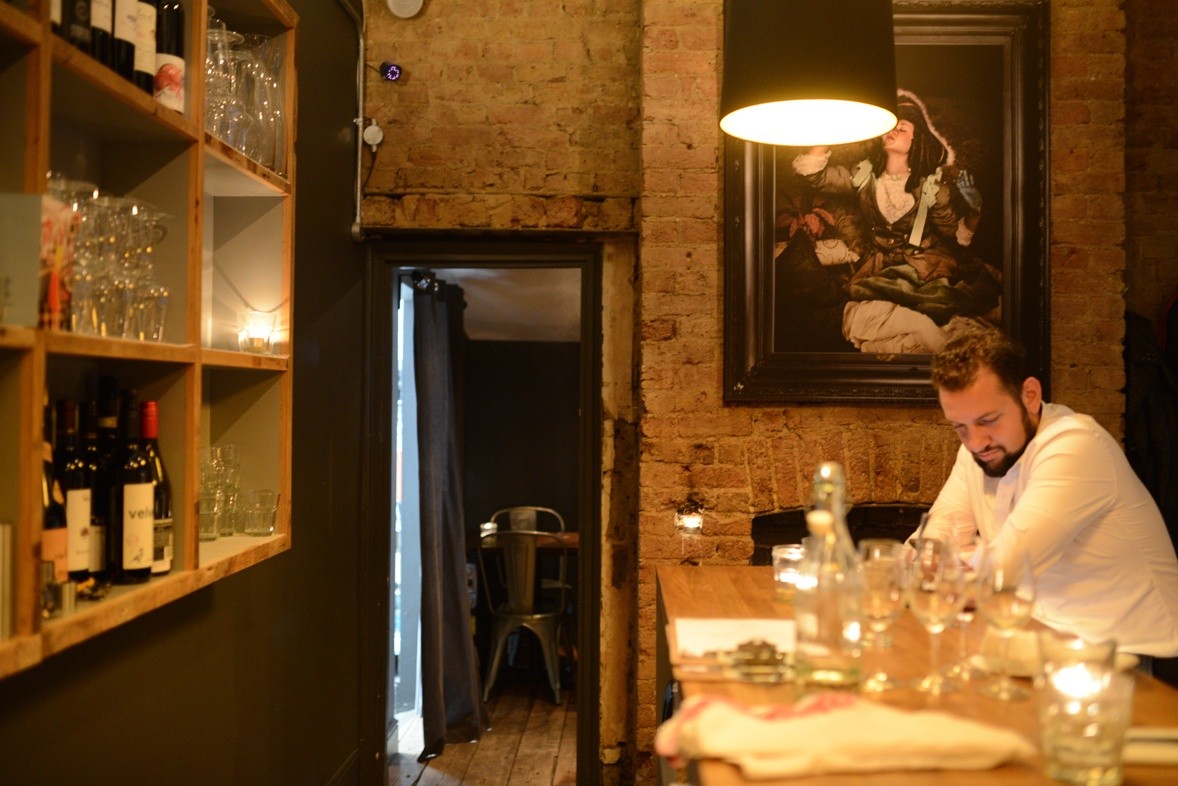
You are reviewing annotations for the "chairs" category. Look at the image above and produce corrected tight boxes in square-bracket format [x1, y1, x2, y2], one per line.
[477, 504, 576, 704]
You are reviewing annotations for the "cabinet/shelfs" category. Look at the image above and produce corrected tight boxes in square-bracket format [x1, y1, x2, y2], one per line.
[0, 1, 300, 681]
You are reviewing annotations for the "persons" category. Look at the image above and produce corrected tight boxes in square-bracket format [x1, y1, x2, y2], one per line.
[903, 315, 1178, 690]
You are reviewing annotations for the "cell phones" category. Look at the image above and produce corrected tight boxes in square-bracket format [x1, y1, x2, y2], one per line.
[907, 537, 974, 576]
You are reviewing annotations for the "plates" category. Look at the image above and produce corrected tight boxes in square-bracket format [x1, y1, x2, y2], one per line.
[969, 652, 1140, 678]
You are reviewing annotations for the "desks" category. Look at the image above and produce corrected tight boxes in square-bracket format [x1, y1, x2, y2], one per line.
[465, 527, 579, 554]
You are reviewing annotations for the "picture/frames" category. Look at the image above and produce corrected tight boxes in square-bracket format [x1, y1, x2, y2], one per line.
[723, 0, 1052, 409]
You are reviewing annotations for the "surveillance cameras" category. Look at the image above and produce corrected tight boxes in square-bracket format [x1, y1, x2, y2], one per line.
[408, 269, 437, 294]
[379, 60, 402, 82]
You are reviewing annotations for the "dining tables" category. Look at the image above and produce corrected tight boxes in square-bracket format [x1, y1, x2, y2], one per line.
[647, 563, 1178, 786]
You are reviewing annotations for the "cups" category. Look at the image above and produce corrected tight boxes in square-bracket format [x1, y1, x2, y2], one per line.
[204, 6, 288, 176]
[772, 542, 804, 600]
[236, 309, 281, 356]
[480, 522, 498, 538]
[197, 443, 280, 543]
[1037, 663, 1138, 785]
[1038, 619, 1121, 667]
[38, 168, 166, 344]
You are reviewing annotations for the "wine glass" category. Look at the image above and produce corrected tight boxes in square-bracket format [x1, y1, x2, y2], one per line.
[854, 534, 1035, 704]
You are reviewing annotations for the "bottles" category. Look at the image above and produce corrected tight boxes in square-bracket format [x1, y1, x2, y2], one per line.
[91, 0, 114, 72]
[794, 509, 861, 685]
[153, 0, 187, 115]
[113, 0, 139, 82]
[812, 461, 859, 570]
[131, 0, 159, 96]
[40, 395, 175, 624]
[50, 0, 60, 38]
[60, 0, 91, 55]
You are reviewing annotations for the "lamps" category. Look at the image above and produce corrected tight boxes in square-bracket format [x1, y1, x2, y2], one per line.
[720, 0, 898, 147]
